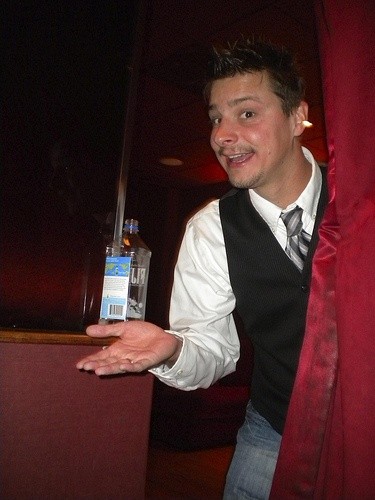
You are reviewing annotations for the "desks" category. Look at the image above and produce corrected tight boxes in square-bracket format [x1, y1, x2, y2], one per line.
[0, 328, 155, 500]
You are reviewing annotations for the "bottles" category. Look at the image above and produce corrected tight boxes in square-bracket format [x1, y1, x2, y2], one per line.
[97, 219, 152, 325]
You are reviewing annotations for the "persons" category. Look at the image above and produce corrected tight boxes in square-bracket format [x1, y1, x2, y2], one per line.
[75, 32, 327, 500]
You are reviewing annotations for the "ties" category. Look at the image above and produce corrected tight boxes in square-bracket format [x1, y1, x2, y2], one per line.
[278, 206, 313, 273]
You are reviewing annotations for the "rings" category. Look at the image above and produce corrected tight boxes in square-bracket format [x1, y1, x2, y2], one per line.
[126, 358, 134, 364]
